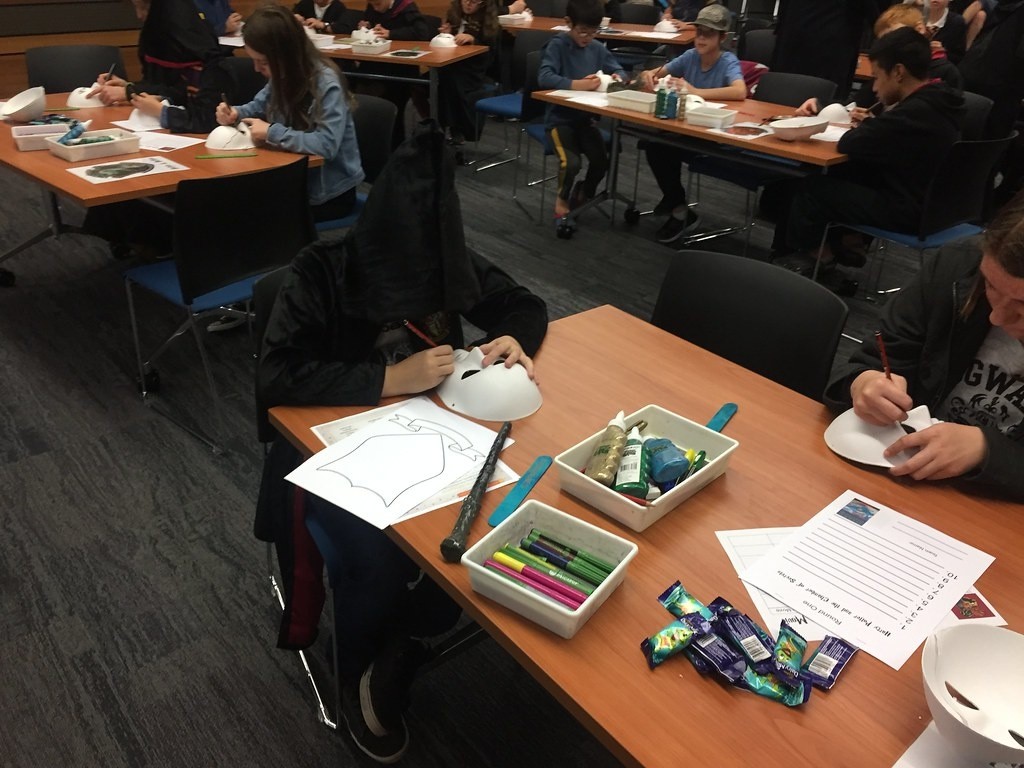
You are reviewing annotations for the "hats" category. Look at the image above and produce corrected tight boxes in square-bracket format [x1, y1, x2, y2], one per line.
[687, 4, 733, 31]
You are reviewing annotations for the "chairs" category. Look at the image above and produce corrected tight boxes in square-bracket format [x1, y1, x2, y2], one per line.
[26, 0, 1024, 731]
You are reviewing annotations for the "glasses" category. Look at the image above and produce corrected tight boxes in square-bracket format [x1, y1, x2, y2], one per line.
[574, 26, 603, 39]
[467, 0, 484, 6]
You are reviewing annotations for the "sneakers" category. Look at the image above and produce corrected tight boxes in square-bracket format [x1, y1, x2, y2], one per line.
[325, 634, 410, 764]
[654, 186, 686, 216]
[655, 208, 703, 243]
[358, 634, 436, 738]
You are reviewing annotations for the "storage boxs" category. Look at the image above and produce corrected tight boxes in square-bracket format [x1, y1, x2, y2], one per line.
[554, 404, 739, 533]
[607, 89, 658, 113]
[11, 124, 70, 151]
[349, 39, 392, 55]
[461, 499, 638, 640]
[306, 34, 336, 47]
[498, 14, 525, 25]
[685, 107, 738, 128]
[45, 127, 141, 163]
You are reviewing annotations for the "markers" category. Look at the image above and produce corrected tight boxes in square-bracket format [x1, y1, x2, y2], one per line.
[501, 543, 597, 595]
[494, 551, 588, 603]
[531, 528, 615, 573]
[68, 135, 120, 146]
[528, 533, 610, 578]
[483, 559, 580, 610]
[221, 93, 230, 109]
[520, 538, 604, 586]
[105, 63, 116, 80]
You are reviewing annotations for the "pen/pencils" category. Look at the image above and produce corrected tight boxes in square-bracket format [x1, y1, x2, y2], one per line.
[738, 111, 755, 116]
[46, 108, 81, 110]
[866, 102, 881, 113]
[196, 154, 258, 158]
[652, 62, 665, 79]
[403, 320, 437, 346]
[758, 116, 773, 126]
[875, 330, 901, 425]
[617, 491, 656, 507]
[577, 78, 593, 79]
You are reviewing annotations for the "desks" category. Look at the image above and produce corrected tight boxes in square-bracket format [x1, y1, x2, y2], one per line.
[267, 304, 1024, 768]
[531, 89, 859, 297]
[217, 31, 489, 133]
[0, 93, 324, 391]
[853, 53, 875, 83]
[498, 17, 697, 65]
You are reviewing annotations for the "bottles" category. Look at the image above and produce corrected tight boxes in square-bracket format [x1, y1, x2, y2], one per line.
[617, 426, 650, 500]
[583, 410, 628, 485]
[57, 119, 93, 145]
[654, 78, 688, 121]
[640, 433, 690, 484]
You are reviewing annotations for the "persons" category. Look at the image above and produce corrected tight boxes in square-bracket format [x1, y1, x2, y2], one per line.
[86, 0, 242, 133]
[824, 207, 1024, 500]
[205, 3, 365, 331]
[538, 0, 747, 242]
[773, 0, 1024, 282]
[292, 0, 535, 146]
[255, 119, 549, 765]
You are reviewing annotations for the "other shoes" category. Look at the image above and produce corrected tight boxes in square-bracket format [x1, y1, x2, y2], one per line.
[569, 181, 584, 208]
[779, 251, 835, 278]
[554, 212, 578, 240]
[830, 231, 867, 268]
[207, 314, 248, 333]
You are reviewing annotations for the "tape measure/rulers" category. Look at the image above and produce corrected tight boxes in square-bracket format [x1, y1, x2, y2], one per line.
[705, 403, 738, 433]
[487, 456, 553, 528]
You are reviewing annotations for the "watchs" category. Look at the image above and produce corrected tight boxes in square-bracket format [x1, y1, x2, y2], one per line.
[324, 23, 329, 31]
